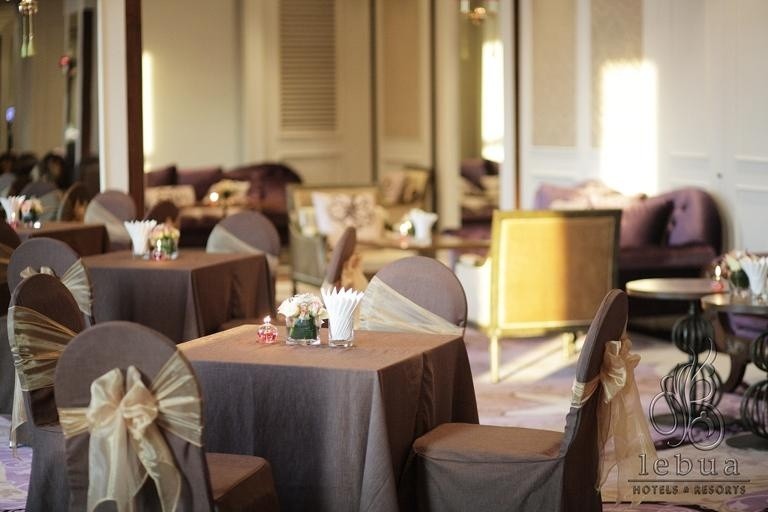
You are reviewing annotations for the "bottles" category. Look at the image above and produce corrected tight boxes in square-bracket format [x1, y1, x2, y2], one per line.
[255, 315, 278, 345]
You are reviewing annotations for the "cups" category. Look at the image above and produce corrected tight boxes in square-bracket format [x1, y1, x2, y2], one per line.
[327, 313, 354, 348]
[131, 237, 150, 262]
[7, 212, 19, 227]
[749, 277, 768, 307]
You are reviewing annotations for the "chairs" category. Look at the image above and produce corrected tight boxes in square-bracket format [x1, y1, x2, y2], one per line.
[1, 148, 767, 512]
